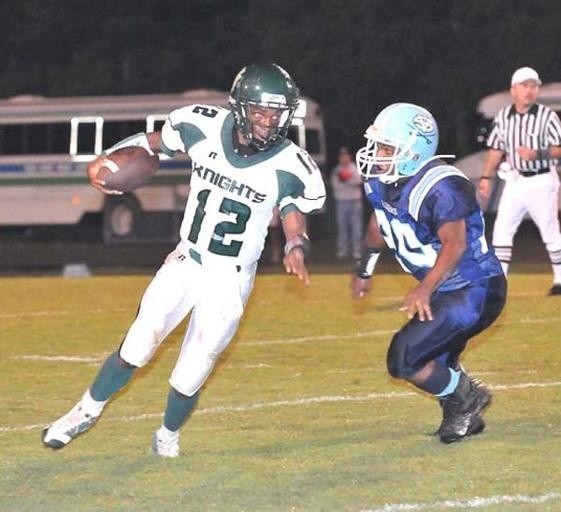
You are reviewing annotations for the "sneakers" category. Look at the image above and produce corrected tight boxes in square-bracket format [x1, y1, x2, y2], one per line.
[42, 401, 100, 449]
[151, 431, 182, 459]
[547, 283, 561, 296]
[429, 416, 485, 440]
[439, 376, 492, 444]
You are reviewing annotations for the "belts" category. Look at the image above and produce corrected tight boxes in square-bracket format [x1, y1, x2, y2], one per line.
[510, 167, 550, 178]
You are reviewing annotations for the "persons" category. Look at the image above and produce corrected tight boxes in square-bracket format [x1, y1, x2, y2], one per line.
[349, 100, 506, 444]
[42, 65, 327, 458]
[474, 66, 561, 297]
[331, 147, 364, 260]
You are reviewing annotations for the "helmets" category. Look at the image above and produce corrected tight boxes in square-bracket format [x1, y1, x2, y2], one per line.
[356, 102, 440, 181]
[228, 62, 300, 152]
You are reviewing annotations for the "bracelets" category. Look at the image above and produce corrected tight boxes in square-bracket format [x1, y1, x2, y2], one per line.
[535, 149, 552, 159]
[284, 233, 311, 256]
[481, 176, 490, 179]
[358, 247, 383, 279]
[103, 132, 153, 161]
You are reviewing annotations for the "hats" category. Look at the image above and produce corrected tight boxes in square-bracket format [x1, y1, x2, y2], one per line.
[511, 67, 543, 87]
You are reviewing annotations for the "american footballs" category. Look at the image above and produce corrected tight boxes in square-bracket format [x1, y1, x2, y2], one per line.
[96, 146, 159, 193]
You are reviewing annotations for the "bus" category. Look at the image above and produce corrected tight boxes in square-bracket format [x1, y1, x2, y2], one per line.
[0, 81, 330, 245]
[470, 80, 560, 155]
